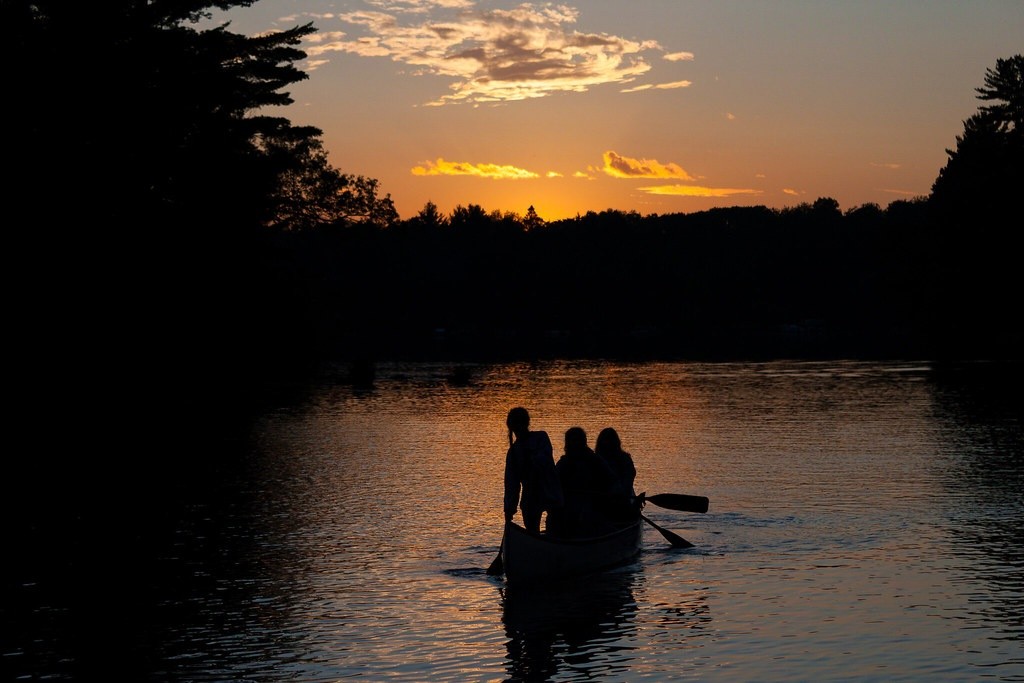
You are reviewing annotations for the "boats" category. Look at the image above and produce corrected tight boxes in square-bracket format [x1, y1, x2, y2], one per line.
[498, 491, 645, 579]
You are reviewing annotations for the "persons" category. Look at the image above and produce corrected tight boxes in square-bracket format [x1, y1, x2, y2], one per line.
[504, 408, 636, 543]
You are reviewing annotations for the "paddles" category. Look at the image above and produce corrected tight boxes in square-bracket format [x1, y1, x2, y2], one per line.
[485, 428, 516, 576]
[641, 512, 697, 547]
[645, 490, 710, 514]
